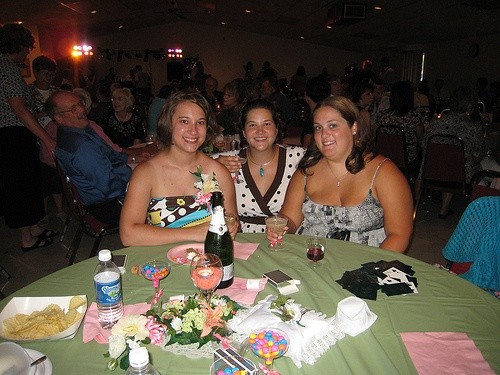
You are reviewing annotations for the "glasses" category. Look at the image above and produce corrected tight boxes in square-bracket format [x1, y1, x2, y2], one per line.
[54, 100, 87, 115]
[111, 96, 130, 101]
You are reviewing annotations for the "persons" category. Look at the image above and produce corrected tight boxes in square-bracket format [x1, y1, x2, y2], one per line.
[119, 91, 240, 246]
[148, 61, 341, 149]
[266, 96, 413, 254]
[0, 24, 57, 252]
[209, 99, 315, 233]
[28, 55, 149, 231]
[346, 77, 500, 219]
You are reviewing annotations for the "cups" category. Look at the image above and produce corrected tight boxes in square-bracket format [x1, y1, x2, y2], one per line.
[209, 356, 256, 375]
[225, 133, 235, 151]
[148, 131, 157, 141]
[0, 342, 37, 375]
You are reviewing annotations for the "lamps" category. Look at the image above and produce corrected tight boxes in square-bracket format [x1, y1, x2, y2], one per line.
[106, 48, 183, 62]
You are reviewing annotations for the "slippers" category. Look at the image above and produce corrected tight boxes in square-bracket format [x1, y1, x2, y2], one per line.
[37, 228, 60, 237]
[439, 208, 454, 219]
[22, 236, 53, 252]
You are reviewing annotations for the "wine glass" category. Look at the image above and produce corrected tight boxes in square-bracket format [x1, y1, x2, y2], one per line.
[139, 261, 171, 305]
[190, 253, 224, 304]
[249, 327, 291, 372]
[265, 216, 287, 250]
[305, 238, 326, 269]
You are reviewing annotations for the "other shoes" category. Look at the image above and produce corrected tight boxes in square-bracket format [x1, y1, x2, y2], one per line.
[40, 215, 50, 226]
[56, 208, 65, 218]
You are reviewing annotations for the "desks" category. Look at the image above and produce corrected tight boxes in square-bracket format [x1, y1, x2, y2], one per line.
[0, 233, 500, 375]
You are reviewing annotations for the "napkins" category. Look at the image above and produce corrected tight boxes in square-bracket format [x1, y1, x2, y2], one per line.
[233, 241, 260, 260]
[82, 302, 150, 344]
[215, 277, 268, 304]
[401, 332, 497, 375]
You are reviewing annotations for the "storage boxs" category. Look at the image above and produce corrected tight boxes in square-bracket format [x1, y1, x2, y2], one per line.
[263, 270, 301, 295]
[111, 254, 128, 274]
[214, 348, 258, 375]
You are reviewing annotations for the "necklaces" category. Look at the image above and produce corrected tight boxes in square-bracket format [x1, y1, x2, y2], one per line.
[337, 171, 349, 187]
[247, 147, 275, 177]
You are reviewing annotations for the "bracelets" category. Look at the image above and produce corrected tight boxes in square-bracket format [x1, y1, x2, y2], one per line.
[131, 153, 136, 164]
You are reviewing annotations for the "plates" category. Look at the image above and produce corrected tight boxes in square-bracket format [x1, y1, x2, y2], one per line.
[166, 243, 204, 265]
[24, 348, 53, 375]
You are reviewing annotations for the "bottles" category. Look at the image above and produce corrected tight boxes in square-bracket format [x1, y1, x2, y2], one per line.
[124, 347, 160, 375]
[94, 250, 123, 325]
[203, 192, 235, 288]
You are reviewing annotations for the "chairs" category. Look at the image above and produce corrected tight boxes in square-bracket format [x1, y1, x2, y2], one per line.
[280, 101, 500, 223]
[51, 151, 120, 267]
[443, 196, 500, 298]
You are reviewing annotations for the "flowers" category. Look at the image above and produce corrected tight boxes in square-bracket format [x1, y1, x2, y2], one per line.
[188, 165, 220, 215]
[103, 315, 150, 371]
[269, 293, 301, 327]
[140, 293, 247, 372]
